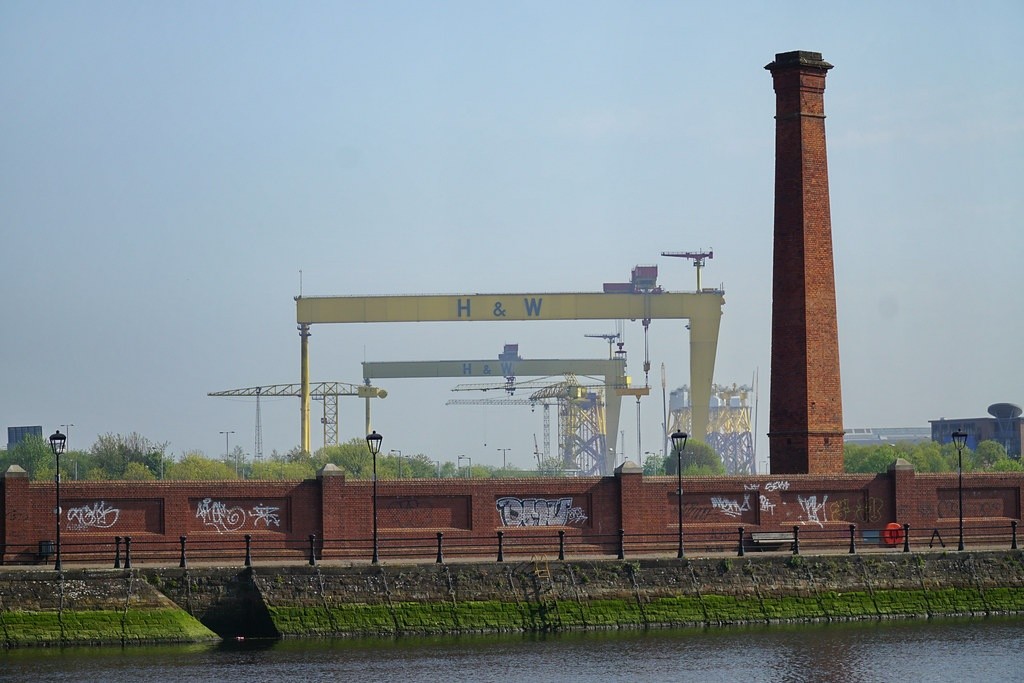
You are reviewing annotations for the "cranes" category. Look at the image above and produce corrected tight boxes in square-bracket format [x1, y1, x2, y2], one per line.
[661, 248, 713, 293]
[533, 434, 540, 465]
[445, 372, 629, 476]
[584, 333, 620, 360]
[207, 378, 388, 449]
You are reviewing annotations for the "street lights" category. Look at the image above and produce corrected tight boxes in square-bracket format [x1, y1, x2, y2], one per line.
[609, 448, 616, 469]
[281, 454, 292, 479]
[49, 430, 66, 570]
[160, 448, 165, 479]
[459, 457, 471, 466]
[243, 453, 250, 479]
[458, 455, 464, 466]
[672, 429, 687, 557]
[391, 449, 401, 478]
[534, 453, 543, 465]
[229, 454, 237, 478]
[645, 452, 656, 475]
[497, 449, 511, 466]
[366, 430, 383, 563]
[952, 429, 968, 551]
[61, 424, 74, 451]
[220, 431, 235, 460]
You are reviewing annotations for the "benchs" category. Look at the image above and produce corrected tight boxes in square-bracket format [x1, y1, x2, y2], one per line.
[752, 530, 801, 553]
